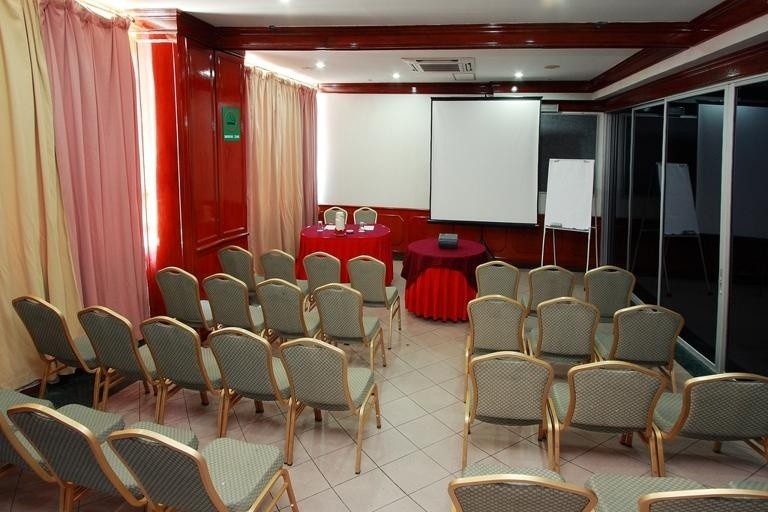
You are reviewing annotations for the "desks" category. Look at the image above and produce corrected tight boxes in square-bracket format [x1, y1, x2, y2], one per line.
[400, 239, 487, 322]
[295, 223, 394, 286]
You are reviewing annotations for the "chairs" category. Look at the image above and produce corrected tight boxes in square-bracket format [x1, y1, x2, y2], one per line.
[448, 466, 598, 512]
[155, 266, 212, 334]
[353, 206, 377, 224]
[463, 294, 527, 403]
[651, 372, 768, 476]
[594, 304, 685, 393]
[312, 283, 387, 371]
[216, 245, 264, 297]
[107, 428, 299, 512]
[549, 360, 659, 477]
[280, 338, 381, 474]
[76, 305, 173, 425]
[584, 265, 636, 320]
[0, 387, 55, 432]
[521, 265, 576, 319]
[12, 295, 105, 410]
[584, 475, 768, 512]
[527, 296, 600, 377]
[303, 251, 340, 312]
[259, 248, 309, 313]
[346, 254, 402, 350]
[324, 207, 348, 224]
[203, 272, 265, 339]
[0, 403, 126, 512]
[138, 316, 224, 438]
[474, 260, 519, 300]
[208, 327, 290, 465]
[255, 278, 320, 343]
[461, 351, 555, 470]
[6, 402, 199, 512]
[729, 478, 768, 491]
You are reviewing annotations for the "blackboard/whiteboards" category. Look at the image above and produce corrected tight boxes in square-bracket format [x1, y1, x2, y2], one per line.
[314, 90, 431, 211]
[546, 158, 596, 233]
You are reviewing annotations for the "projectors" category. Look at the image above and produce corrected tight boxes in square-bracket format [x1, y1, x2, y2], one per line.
[437, 233, 459, 250]
